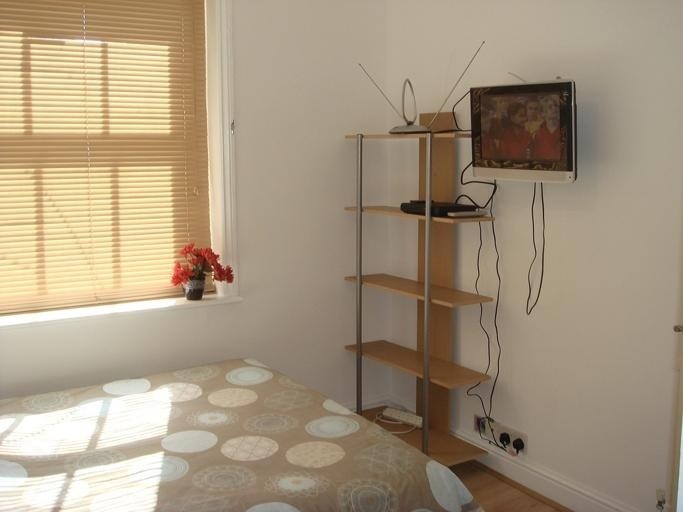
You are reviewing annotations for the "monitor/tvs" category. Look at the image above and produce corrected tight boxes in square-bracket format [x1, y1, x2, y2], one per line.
[469, 80, 577, 184]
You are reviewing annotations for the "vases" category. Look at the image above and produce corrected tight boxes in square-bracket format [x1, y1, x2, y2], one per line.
[183, 279, 205, 300]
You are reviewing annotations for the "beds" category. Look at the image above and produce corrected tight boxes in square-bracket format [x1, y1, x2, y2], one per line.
[0, 358, 484, 512]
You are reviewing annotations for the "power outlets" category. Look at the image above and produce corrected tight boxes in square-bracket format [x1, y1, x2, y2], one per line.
[500, 424, 527, 453]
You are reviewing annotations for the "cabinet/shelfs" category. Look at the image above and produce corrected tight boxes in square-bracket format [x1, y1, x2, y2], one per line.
[345, 131, 495, 468]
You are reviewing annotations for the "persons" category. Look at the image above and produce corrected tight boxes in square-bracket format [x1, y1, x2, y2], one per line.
[480, 92, 561, 160]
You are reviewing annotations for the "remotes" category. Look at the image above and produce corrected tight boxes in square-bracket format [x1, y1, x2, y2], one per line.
[447, 210, 488, 217]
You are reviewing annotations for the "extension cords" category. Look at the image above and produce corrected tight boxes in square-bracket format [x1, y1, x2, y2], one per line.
[382, 407, 423, 429]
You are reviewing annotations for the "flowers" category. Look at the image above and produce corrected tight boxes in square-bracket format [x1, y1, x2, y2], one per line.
[171, 243, 234, 287]
